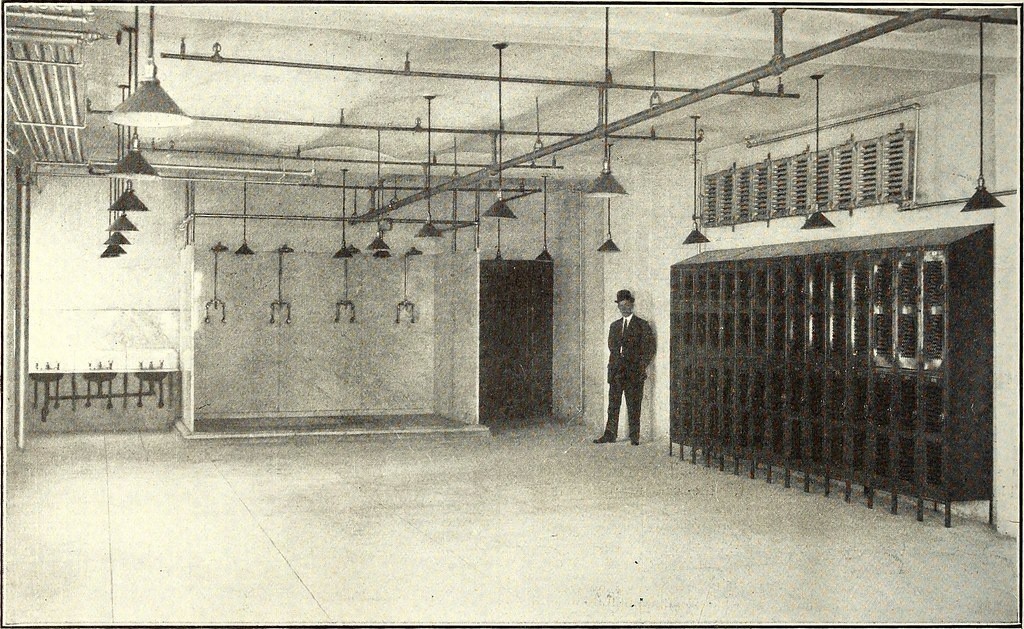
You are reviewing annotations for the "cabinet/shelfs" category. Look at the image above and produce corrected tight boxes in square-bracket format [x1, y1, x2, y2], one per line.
[667, 222, 996, 532]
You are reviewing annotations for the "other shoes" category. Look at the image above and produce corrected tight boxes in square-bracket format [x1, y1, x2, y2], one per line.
[593, 434, 616, 443]
[630, 437, 640, 446]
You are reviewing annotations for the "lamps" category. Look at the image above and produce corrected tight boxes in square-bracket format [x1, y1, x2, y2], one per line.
[95, 7, 1006, 259]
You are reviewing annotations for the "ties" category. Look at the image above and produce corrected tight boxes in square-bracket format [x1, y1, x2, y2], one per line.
[621, 319, 627, 355]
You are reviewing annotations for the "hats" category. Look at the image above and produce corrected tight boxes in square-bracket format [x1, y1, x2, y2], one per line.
[614, 290, 635, 303]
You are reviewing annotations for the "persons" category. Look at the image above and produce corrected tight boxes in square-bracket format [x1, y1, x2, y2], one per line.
[592, 290, 656, 445]
